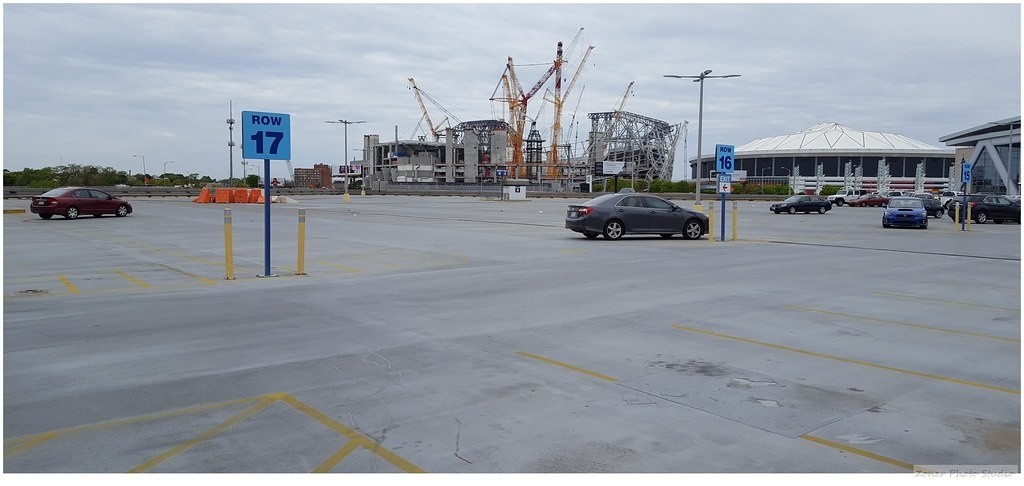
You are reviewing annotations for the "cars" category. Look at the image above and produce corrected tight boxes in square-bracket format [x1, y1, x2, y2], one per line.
[941, 190, 964, 209]
[886, 191, 942, 201]
[770, 194, 833, 214]
[29, 186, 133, 221]
[848, 193, 892, 207]
[565, 192, 709, 241]
[882, 196, 929, 229]
[919, 198, 945, 218]
[947, 193, 1021, 224]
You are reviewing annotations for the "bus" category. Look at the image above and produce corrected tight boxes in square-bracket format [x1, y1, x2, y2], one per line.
[709, 170, 748, 185]
[331, 177, 365, 190]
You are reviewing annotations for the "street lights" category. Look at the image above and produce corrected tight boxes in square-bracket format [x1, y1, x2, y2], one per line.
[132, 154, 147, 187]
[761, 166, 772, 194]
[989, 122, 1020, 194]
[781, 166, 791, 195]
[163, 160, 175, 186]
[662, 69, 743, 212]
[252, 163, 260, 189]
[325, 120, 367, 202]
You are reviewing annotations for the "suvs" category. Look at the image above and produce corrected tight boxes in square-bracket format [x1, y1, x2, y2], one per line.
[828, 190, 861, 206]
